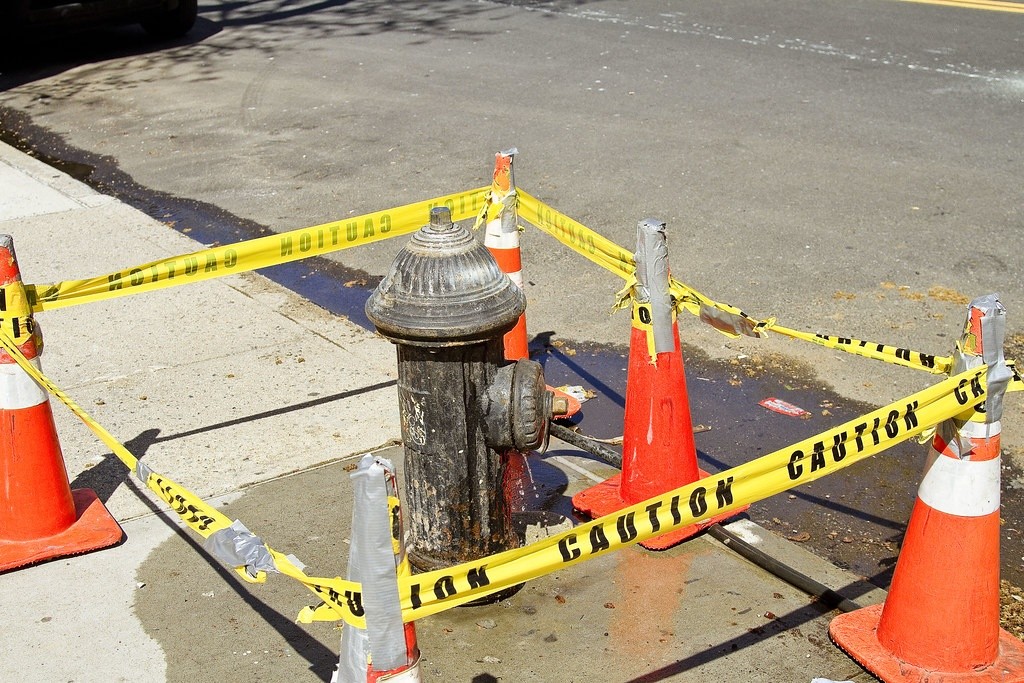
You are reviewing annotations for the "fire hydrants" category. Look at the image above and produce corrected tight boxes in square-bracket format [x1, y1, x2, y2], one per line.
[365, 205, 570, 610]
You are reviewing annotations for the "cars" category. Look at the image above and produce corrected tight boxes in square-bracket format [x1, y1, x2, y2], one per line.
[0, 0, 200, 67]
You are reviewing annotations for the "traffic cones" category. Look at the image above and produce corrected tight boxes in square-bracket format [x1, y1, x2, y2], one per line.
[569, 216, 751, 553]
[334, 454, 424, 683]
[0, 229, 126, 571]
[824, 296, 1024, 683]
[481, 151, 582, 420]
